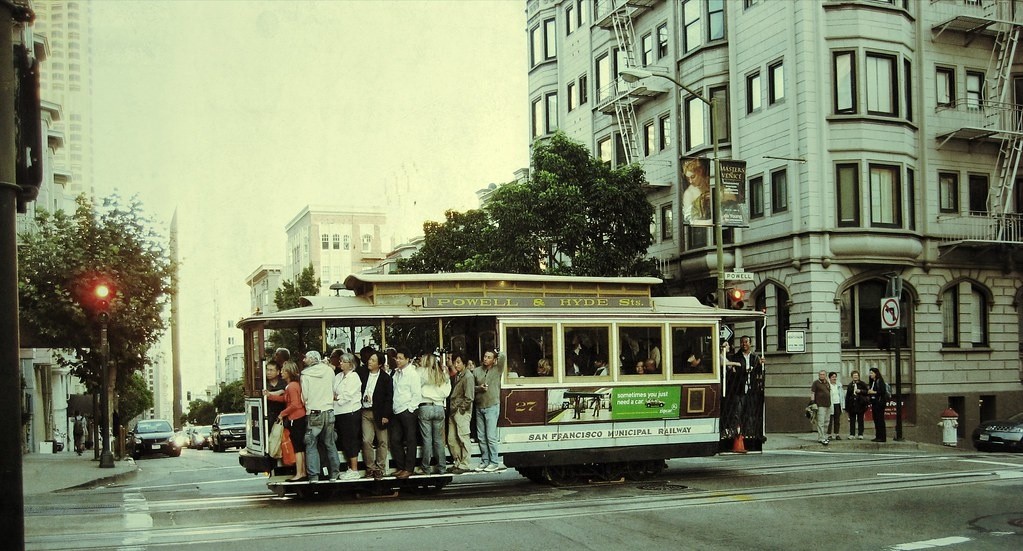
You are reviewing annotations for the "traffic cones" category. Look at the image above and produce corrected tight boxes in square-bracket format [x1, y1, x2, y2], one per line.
[730, 422, 747, 453]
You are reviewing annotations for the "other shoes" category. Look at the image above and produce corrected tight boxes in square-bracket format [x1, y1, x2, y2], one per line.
[445, 465, 471, 474]
[284, 475, 309, 482]
[374, 470, 384, 480]
[858, 435, 864, 440]
[817, 439, 830, 445]
[415, 468, 430, 475]
[827, 435, 832, 440]
[329, 474, 337, 481]
[390, 469, 403, 476]
[395, 469, 414, 479]
[871, 437, 886, 442]
[836, 435, 841, 440]
[847, 434, 856, 440]
[309, 475, 318, 483]
[365, 470, 374, 479]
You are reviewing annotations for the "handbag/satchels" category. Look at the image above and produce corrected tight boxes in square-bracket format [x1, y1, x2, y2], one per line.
[267, 418, 297, 467]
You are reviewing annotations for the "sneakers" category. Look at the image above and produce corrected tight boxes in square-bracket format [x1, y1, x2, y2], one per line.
[338, 466, 361, 480]
[474, 464, 487, 472]
[483, 464, 499, 472]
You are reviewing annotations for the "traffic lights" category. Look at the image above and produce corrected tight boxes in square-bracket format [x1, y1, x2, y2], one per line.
[94, 286, 110, 324]
[731, 289, 744, 310]
[707, 293, 718, 308]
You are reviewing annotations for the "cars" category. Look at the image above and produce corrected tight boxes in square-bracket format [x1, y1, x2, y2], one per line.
[175, 426, 212, 451]
[125, 419, 181, 460]
[972, 412, 1023, 453]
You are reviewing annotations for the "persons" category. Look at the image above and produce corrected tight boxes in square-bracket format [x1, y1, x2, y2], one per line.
[845, 371, 869, 440]
[687, 352, 707, 372]
[568, 334, 608, 376]
[721, 336, 767, 442]
[806, 370, 831, 445]
[261, 348, 505, 484]
[70, 410, 87, 456]
[828, 372, 845, 440]
[621, 332, 661, 374]
[856, 368, 887, 442]
[508, 333, 551, 377]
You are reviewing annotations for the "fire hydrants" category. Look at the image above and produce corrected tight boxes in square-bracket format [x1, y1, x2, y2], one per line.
[937, 408, 959, 447]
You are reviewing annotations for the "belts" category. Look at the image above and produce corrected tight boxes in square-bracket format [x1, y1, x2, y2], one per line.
[418, 402, 434, 407]
[361, 407, 372, 411]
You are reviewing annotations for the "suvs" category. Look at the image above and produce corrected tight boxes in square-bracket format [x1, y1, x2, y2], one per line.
[210, 412, 246, 452]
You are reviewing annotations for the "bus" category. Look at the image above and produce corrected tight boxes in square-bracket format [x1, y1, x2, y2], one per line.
[238, 271, 768, 501]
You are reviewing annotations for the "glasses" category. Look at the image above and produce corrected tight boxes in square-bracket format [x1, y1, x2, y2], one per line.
[339, 359, 351, 363]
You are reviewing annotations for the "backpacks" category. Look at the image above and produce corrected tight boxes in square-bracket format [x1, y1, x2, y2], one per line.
[876, 378, 893, 401]
[72, 416, 85, 439]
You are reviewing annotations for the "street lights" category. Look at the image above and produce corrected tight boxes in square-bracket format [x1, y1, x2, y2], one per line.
[620, 68, 725, 308]
